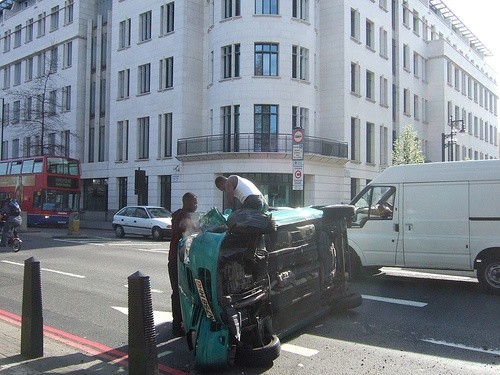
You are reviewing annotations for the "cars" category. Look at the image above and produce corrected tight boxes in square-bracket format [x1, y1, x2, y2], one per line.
[179, 207, 364, 365]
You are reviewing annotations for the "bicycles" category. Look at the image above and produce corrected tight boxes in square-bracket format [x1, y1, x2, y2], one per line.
[0, 212, 21, 257]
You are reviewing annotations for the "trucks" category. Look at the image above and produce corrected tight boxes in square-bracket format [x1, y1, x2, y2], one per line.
[341, 160, 500, 293]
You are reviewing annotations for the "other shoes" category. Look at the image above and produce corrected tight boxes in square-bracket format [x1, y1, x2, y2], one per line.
[172, 325, 184, 336]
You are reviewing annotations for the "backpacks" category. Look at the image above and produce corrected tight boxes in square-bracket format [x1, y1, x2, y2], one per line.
[6, 200, 21, 216]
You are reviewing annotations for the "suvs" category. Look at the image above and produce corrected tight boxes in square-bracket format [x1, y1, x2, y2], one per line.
[110, 206, 176, 241]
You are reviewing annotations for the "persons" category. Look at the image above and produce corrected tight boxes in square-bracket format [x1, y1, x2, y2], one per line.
[215, 175, 268, 213]
[168, 192, 197, 337]
[0, 197, 22, 246]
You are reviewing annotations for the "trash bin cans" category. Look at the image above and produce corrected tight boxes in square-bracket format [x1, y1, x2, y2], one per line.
[68, 212, 80, 235]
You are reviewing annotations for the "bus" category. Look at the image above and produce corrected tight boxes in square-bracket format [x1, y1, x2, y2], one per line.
[0, 157, 83, 229]
